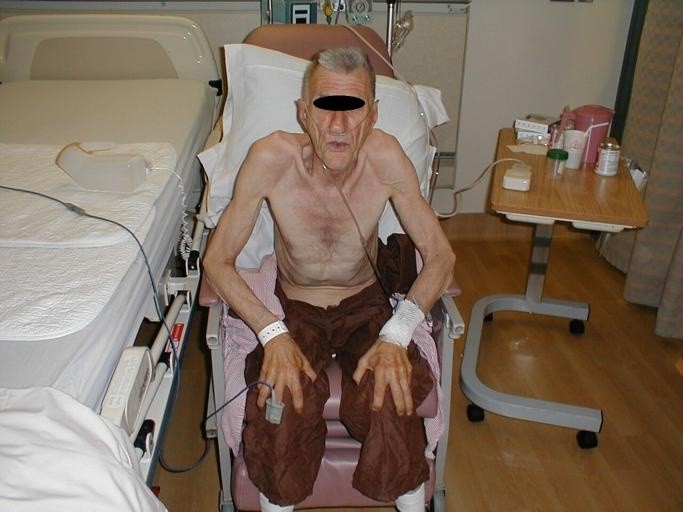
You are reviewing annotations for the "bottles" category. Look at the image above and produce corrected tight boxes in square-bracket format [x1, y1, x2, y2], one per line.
[542, 149, 568, 184]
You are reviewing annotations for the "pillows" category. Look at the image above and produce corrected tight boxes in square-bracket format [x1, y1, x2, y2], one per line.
[212, 37, 442, 217]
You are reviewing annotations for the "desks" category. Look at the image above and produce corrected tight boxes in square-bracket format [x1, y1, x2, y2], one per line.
[457, 121, 649, 451]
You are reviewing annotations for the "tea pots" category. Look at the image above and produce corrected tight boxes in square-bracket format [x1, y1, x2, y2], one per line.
[554, 104, 617, 170]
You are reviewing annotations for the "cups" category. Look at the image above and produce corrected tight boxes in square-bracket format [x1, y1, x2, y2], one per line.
[563, 130, 588, 169]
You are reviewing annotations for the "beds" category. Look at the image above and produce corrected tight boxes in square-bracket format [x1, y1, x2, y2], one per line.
[1, 9, 221, 503]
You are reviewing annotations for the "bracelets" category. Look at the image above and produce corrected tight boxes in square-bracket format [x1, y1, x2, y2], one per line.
[379, 301, 424, 348]
[257, 320, 288, 347]
